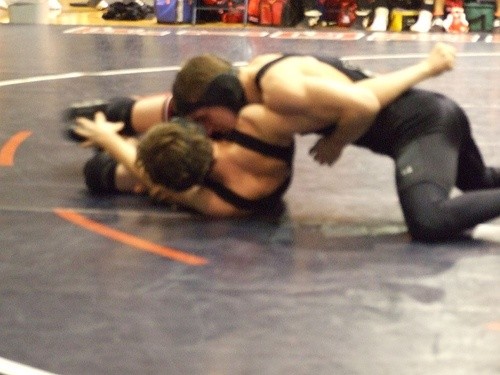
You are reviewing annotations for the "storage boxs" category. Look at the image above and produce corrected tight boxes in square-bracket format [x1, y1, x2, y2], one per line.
[462, 3, 496, 32]
[153, 0, 194, 24]
[244, 0, 295, 27]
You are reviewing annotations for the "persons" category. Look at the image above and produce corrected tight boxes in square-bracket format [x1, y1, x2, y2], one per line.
[59, 38, 459, 221]
[171, 48, 500, 245]
[302, 0, 471, 33]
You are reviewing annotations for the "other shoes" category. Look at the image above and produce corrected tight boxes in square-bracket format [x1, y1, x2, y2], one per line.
[100, 1, 151, 20]
[366, 6, 389, 31]
[409, 9, 432, 32]
[428, 13, 445, 32]
[64, 95, 112, 143]
